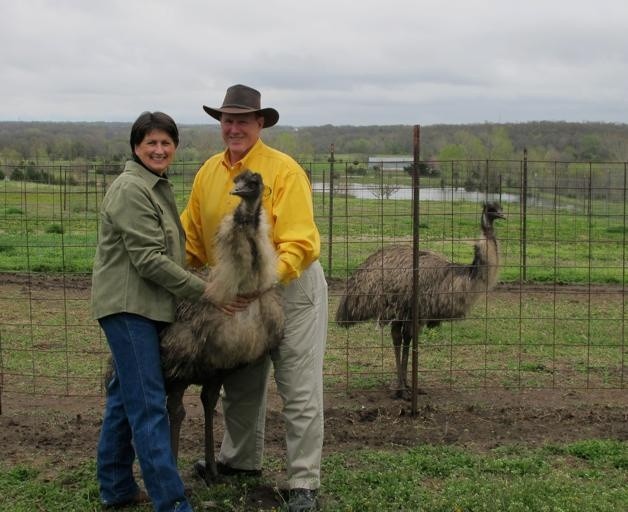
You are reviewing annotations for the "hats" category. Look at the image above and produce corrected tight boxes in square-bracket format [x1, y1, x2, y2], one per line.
[202, 84, 280, 129]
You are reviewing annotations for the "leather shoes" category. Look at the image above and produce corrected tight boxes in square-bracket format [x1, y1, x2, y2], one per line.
[287, 488, 321, 512]
[194, 459, 263, 480]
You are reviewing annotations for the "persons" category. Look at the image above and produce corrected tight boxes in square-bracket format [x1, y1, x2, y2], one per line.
[90, 111, 250, 511]
[178, 83, 329, 512]
